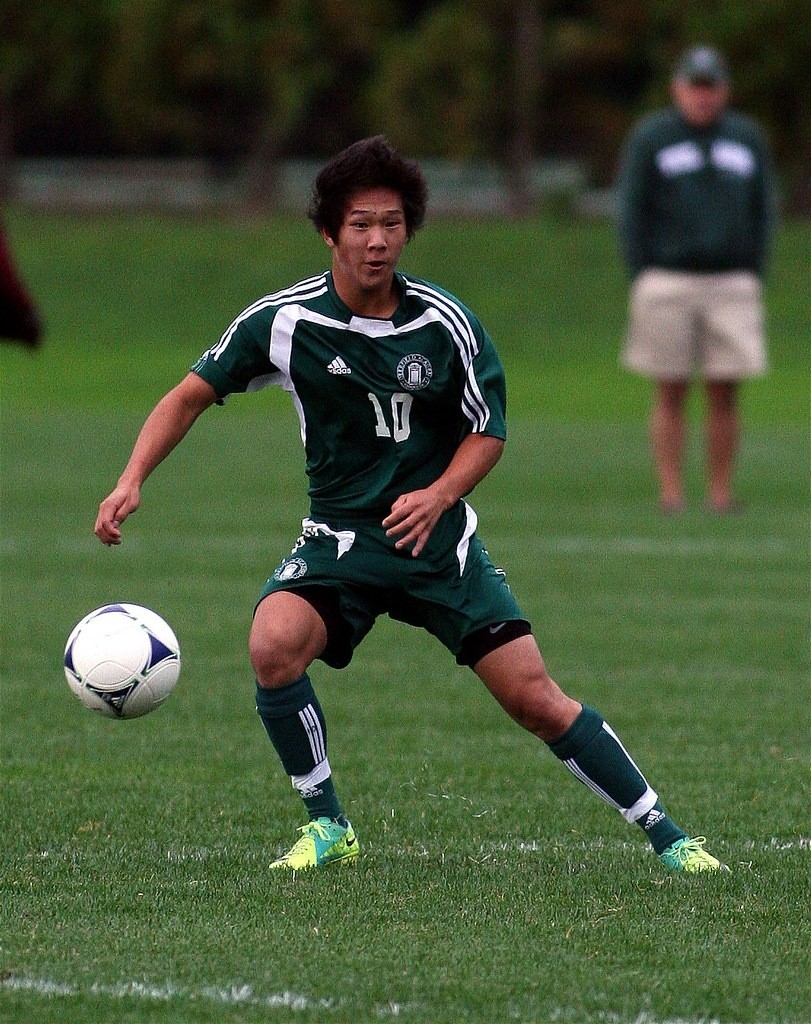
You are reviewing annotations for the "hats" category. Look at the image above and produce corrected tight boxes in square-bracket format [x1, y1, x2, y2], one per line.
[673, 43, 737, 82]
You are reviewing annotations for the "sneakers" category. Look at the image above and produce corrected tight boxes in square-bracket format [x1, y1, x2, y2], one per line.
[267, 815, 360, 872]
[658, 838, 734, 874]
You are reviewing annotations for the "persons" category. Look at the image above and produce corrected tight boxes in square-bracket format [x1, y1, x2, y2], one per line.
[92, 132, 737, 878]
[615, 45, 774, 512]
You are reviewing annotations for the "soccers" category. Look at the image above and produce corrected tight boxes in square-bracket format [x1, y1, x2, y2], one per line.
[62, 603, 182, 718]
